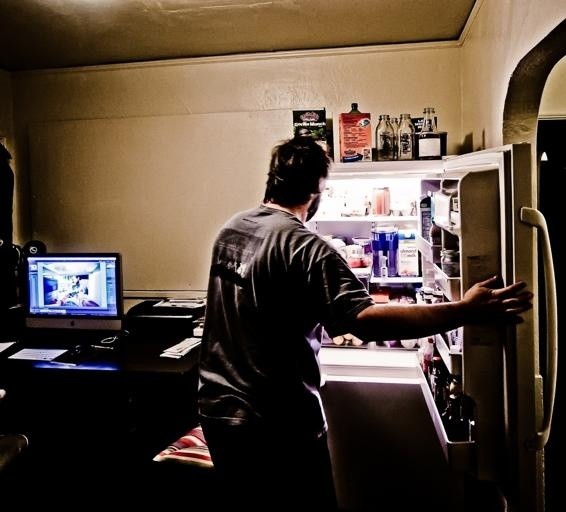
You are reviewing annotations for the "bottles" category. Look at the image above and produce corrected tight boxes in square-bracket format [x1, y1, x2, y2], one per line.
[374, 105, 439, 161]
[421, 338, 470, 443]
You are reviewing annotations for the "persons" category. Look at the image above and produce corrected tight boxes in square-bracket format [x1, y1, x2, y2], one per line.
[196, 138, 534, 511]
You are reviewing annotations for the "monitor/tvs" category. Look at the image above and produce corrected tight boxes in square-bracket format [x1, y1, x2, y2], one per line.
[25, 253, 124, 331]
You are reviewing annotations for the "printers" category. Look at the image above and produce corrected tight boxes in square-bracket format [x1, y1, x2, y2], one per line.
[125, 298, 207, 364]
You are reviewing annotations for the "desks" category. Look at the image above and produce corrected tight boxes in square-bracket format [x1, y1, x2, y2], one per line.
[0, 326, 204, 512]
